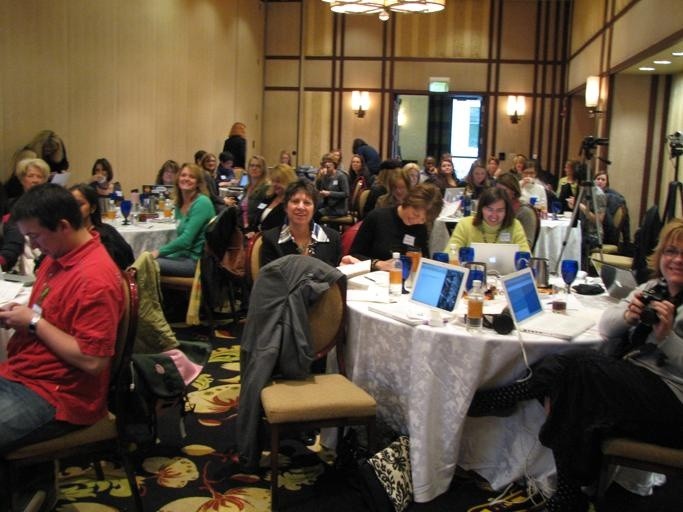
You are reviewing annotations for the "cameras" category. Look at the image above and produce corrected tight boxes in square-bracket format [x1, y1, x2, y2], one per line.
[578, 136, 612, 164]
[639, 289, 680, 327]
[668, 131, 683, 143]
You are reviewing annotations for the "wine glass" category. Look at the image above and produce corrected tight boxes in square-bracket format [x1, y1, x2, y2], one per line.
[120, 199, 132, 225]
[458, 194, 562, 221]
[401, 248, 580, 298]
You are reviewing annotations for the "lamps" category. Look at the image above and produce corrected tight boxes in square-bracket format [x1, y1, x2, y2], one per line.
[585, 74, 611, 117]
[350, 89, 372, 118]
[506, 95, 529, 125]
[320, 0, 446, 21]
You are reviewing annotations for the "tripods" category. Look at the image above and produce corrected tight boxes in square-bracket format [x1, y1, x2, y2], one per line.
[554, 156, 613, 276]
[662, 156, 683, 226]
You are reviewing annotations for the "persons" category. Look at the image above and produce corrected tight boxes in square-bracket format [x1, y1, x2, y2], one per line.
[254, 123, 683, 511]
[0, 122, 268, 443]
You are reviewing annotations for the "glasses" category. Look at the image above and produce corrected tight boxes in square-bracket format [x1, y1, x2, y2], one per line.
[661, 244, 682, 258]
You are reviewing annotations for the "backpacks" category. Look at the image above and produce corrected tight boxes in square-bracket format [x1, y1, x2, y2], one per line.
[125, 350, 186, 453]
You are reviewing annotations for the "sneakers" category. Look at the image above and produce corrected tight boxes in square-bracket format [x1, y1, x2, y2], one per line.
[466, 389, 517, 418]
[44, 469, 59, 510]
[17, 487, 47, 511]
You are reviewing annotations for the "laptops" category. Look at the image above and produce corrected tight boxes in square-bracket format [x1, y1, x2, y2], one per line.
[368, 257, 470, 326]
[499, 266, 597, 340]
[228, 173, 250, 192]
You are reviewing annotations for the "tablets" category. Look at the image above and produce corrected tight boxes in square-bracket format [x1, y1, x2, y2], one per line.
[470, 242, 519, 276]
[444, 187, 465, 202]
[591, 259, 638, 300]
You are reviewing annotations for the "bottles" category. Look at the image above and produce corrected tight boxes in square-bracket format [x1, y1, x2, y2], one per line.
[390, 253, 402, 297]
[465, 279, 484, 328]
[163, 199, 171, 217]
[158, 193, 165, 211]
[106, 199, 117, 225]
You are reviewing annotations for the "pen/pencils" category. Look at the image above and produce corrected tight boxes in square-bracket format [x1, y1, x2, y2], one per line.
[37, 287, 50, 306]
[358, 275, 376, 280]
